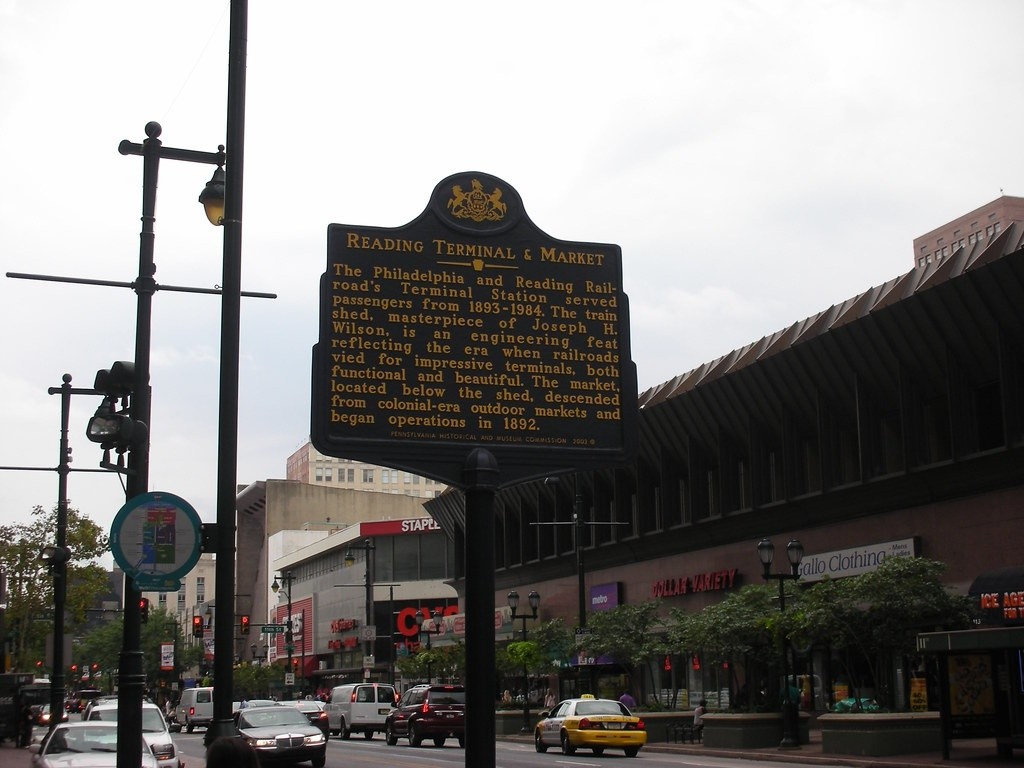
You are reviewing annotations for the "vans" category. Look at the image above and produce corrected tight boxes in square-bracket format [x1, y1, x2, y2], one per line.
[176, 687, 215, 733]
[325, 683, 401, 741]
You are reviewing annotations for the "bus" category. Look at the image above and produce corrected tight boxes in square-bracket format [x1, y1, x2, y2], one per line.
[78, 689, 102, 706]
[502, 663, 633, 704]
[18, 681, 68, 719]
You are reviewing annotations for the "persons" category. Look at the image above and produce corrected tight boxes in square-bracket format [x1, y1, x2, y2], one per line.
[313, 687, 330, 702]
[165, 696, 181, 727]
[543, 687, 556, 709]
[850, 701, 868, 714]
[694, 700, 708, 737]
[619, 689, 636, 712]
[21, 701, 34, 750]
[206, 736, 260, 768]
[269, 693, 276, 701]
[499, 686, 523, 703]
[83, 699, 99, 721]
[238, 696, 248, 709]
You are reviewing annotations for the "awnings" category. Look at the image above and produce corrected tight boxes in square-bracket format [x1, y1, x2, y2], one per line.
[276, 655, 320, 678]
[967, 565, 1024, 626]
[638, 616, 678, 634]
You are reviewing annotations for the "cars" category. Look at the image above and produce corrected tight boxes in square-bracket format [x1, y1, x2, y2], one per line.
[787, 675, 822, 700]
[234, 699, 329, 767]
[533, 693, 647, 759]
[28, 697, 186, 768]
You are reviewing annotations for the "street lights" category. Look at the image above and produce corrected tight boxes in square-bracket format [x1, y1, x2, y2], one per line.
[415, 609, 442, 684]
[163, 620, 178, 670]
[757, 536, 807, 752]
[272, 570, 292, 672]
[117, 122, 229, 767]
[345, 539, 379, 682]
[507, 589, 542, 732]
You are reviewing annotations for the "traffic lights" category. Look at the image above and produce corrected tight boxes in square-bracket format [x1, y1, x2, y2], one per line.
[241, 615, 249, 635]
[193, 616, 203, 638]
[139, 598, 149, 623]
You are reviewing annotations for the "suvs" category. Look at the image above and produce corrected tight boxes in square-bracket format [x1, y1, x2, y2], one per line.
[385, 684, 466, 747]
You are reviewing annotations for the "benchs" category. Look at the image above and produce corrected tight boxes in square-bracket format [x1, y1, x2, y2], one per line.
[666, 722, 701, 745]
